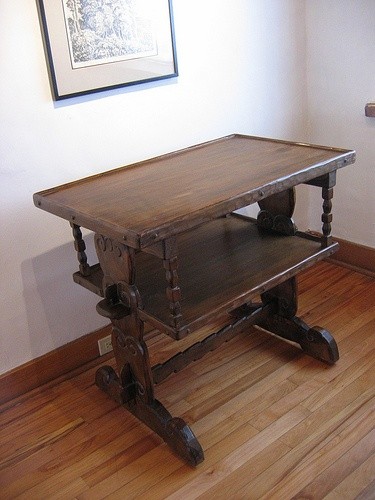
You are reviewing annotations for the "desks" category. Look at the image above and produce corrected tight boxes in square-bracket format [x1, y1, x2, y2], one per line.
[32, 132, 356, 467]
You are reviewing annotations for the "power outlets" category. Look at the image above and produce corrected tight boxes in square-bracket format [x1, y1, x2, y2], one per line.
[98, 334, 114, 356]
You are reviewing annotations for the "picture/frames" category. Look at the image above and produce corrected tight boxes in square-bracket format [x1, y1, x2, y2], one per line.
[35, 0, 179, 101]
